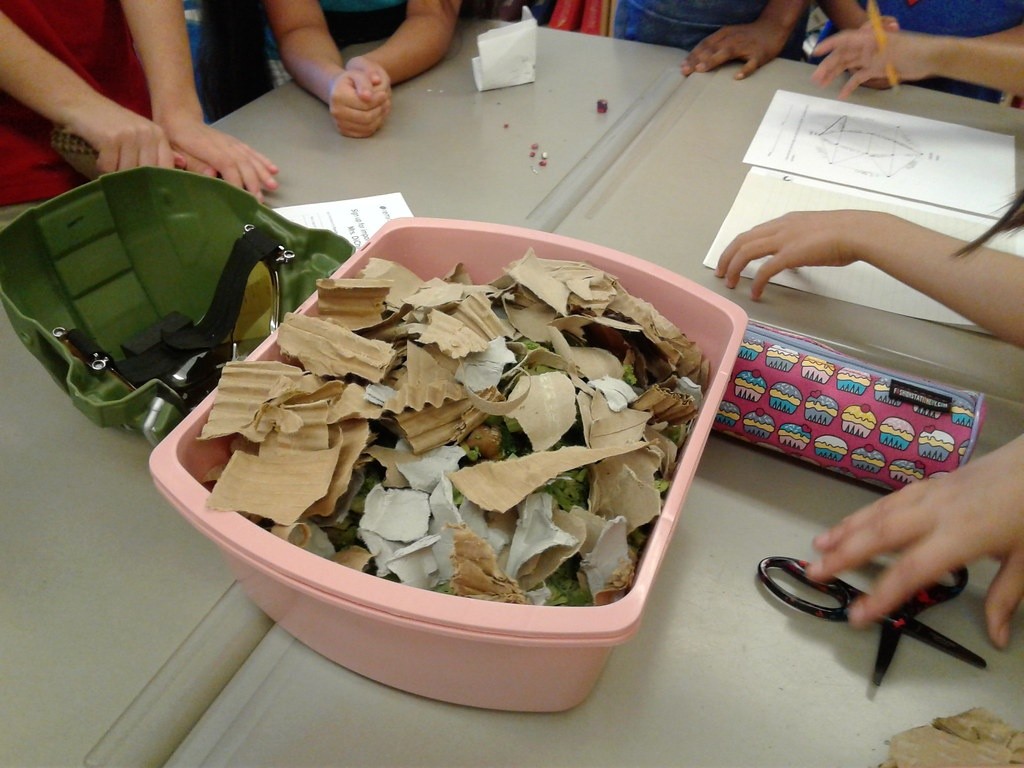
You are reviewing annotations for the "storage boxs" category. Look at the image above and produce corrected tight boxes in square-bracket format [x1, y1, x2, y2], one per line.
[149, 216, 748, 713]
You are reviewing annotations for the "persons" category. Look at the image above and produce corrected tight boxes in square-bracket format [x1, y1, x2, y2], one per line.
[806, 434, 1024, 650]
[0, 1, 279, 206]
[614, 1, 1024, 349]
[271, 0, 458, 137]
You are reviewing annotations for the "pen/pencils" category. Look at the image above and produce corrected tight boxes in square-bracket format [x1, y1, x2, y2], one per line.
[864, 0, 902, 94]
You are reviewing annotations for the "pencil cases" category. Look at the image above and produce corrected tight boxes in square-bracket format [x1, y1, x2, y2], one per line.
[711, 320, 985, 493]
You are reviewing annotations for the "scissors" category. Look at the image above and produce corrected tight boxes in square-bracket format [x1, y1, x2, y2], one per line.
[759, 554, 987, 687]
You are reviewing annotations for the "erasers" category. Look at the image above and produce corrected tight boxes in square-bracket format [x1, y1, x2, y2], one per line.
[597, 99, 608, 114]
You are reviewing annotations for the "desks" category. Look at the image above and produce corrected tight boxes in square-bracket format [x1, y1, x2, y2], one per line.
[0, 19, 691, 768]
[165, 57, 1023, 767]
